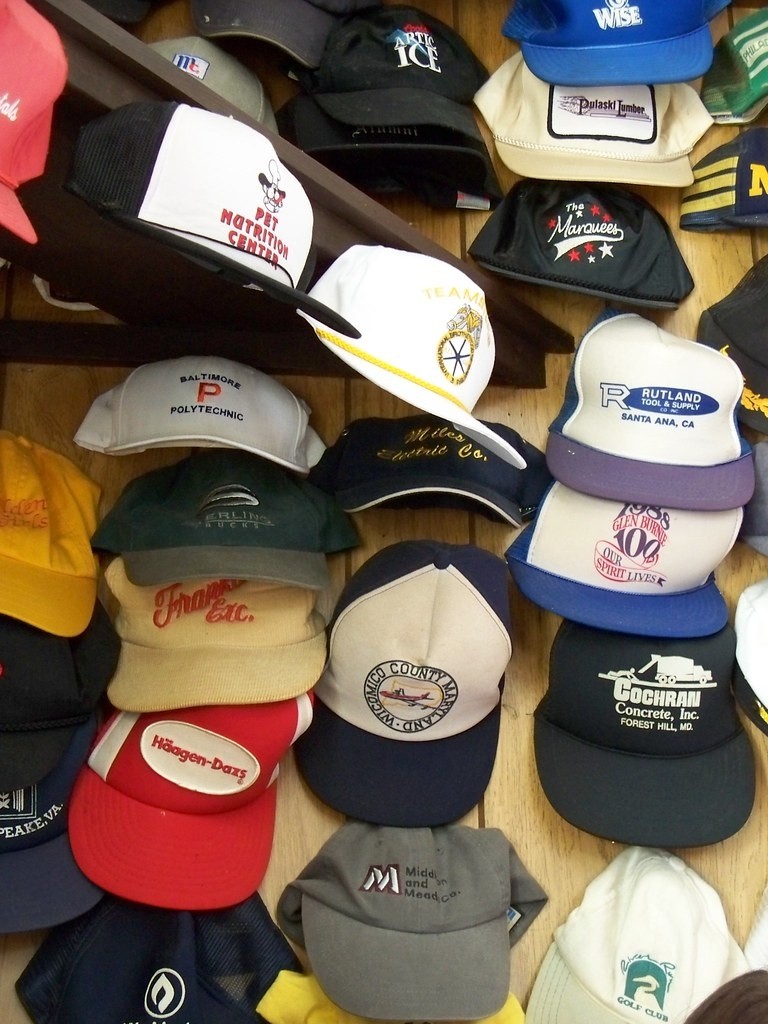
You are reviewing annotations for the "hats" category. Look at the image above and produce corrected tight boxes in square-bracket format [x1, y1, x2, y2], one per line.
[0, 4, 768, 1024]
